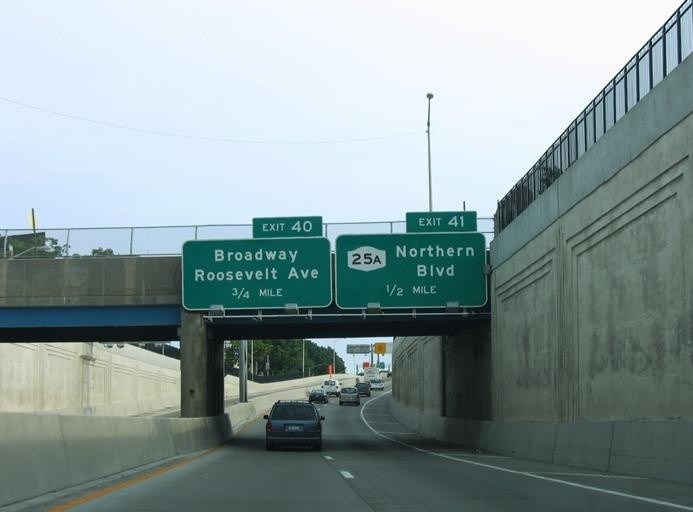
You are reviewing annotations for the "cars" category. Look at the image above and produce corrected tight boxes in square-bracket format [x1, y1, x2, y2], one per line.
[309, 367, 384, 405]
[263, 400, 325, 450]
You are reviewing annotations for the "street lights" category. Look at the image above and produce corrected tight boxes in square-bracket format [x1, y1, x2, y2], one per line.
[427, 93, 433, 211]
